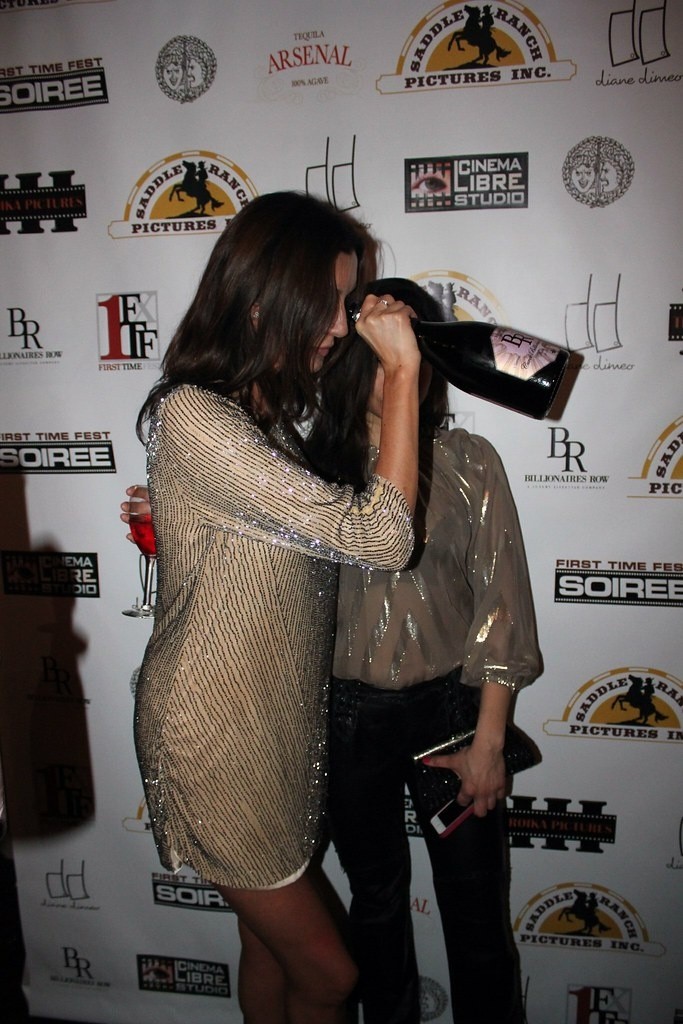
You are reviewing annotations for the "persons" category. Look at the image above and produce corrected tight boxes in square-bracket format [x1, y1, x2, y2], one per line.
[119, 277, 545, 1024]
[132, 192, 421, 1023]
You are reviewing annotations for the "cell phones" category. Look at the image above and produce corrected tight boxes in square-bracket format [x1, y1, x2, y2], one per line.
[429, 795, 476, 838]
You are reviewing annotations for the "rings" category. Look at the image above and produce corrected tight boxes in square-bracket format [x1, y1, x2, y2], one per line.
[352, 309, 361, 322]
[377, 300, 388, 306]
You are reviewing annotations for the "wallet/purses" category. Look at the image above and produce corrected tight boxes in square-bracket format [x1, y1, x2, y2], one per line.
[413, 719, 543, 807]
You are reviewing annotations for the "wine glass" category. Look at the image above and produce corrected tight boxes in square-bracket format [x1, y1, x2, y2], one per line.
[122, 486, 157, 618]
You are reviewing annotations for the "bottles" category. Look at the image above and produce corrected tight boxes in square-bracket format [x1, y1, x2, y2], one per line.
[352, 309, 570, 420]
